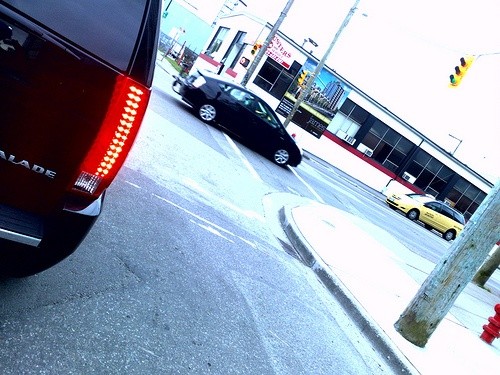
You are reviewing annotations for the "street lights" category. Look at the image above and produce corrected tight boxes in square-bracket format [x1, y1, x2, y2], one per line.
[282, 11, 368, 129]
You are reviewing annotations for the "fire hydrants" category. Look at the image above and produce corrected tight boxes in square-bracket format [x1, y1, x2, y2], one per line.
[479, 303, 500, 344]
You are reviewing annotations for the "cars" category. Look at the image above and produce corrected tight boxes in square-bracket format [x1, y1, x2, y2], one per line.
[178, 73, 303, 168]
[385, 190, 467, 243]
[0, 0, 164, 273]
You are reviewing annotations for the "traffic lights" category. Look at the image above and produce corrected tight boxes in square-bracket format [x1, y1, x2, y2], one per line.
[448, 55, 475, 87]
